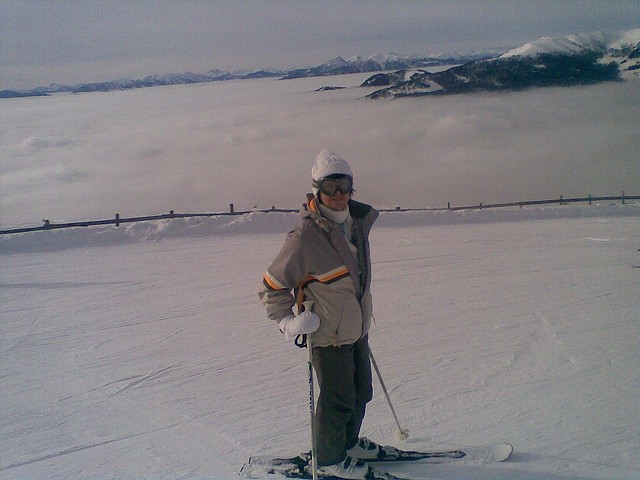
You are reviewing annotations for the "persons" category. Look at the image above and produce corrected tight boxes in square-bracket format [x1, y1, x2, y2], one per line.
[257, 149, 385, 479]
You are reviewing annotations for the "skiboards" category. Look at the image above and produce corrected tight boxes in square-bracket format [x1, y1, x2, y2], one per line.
[238, 444, 513, 479]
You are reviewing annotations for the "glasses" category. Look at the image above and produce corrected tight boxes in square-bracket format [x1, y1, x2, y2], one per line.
[311, 176, 353, 196]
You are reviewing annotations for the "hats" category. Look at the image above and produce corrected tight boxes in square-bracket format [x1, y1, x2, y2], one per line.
[311, 150, 353, 199]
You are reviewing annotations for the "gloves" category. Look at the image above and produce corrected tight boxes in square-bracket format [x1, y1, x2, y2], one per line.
[278, 311, 320, 341]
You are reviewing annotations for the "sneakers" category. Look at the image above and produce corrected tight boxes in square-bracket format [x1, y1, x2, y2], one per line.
[308, 456, 368, 480]
[346, 437, 385, 461]
